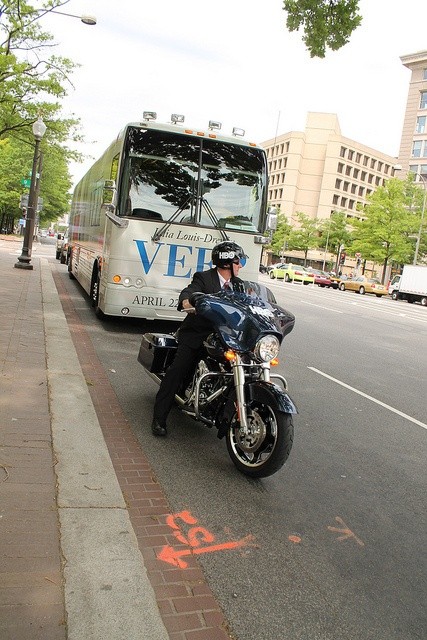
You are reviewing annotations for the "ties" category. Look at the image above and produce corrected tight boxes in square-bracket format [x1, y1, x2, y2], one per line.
[225, 282, 232, 290]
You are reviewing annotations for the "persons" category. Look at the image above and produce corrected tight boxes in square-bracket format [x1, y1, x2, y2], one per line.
[150, 241, 246, 436]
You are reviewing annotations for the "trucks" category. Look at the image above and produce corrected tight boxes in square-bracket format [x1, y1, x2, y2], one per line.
[387, 264, 426, 306]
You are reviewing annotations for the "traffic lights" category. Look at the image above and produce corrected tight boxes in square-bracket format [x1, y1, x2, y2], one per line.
[340, 254, 345, 264]
[356, 258, 360, 265]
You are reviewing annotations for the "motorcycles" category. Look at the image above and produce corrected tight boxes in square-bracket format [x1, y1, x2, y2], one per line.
[136, 281, 299, 478]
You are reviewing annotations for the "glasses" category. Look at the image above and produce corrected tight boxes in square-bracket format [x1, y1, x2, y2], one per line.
[233, 260, 240, 265]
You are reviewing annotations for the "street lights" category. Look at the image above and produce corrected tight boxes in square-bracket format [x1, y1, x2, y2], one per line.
[15, 115, 46, 270]
[391, 165, 426, 265]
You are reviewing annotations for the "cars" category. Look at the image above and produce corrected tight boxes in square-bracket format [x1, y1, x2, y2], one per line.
[268, 263, 314, 285]
[309, 269, 331, 287]
[339, 276, 388, 297]
[329, 274, 341, 289]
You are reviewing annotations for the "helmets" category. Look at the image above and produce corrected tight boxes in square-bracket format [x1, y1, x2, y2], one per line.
[212, 241, 246, 265]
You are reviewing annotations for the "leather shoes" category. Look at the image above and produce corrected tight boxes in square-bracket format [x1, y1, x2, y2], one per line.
[152, 422, 167, 435]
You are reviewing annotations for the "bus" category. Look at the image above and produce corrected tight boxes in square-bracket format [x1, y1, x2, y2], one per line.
[49, 229, 54, 237]
[68, 111, 276, 320]
